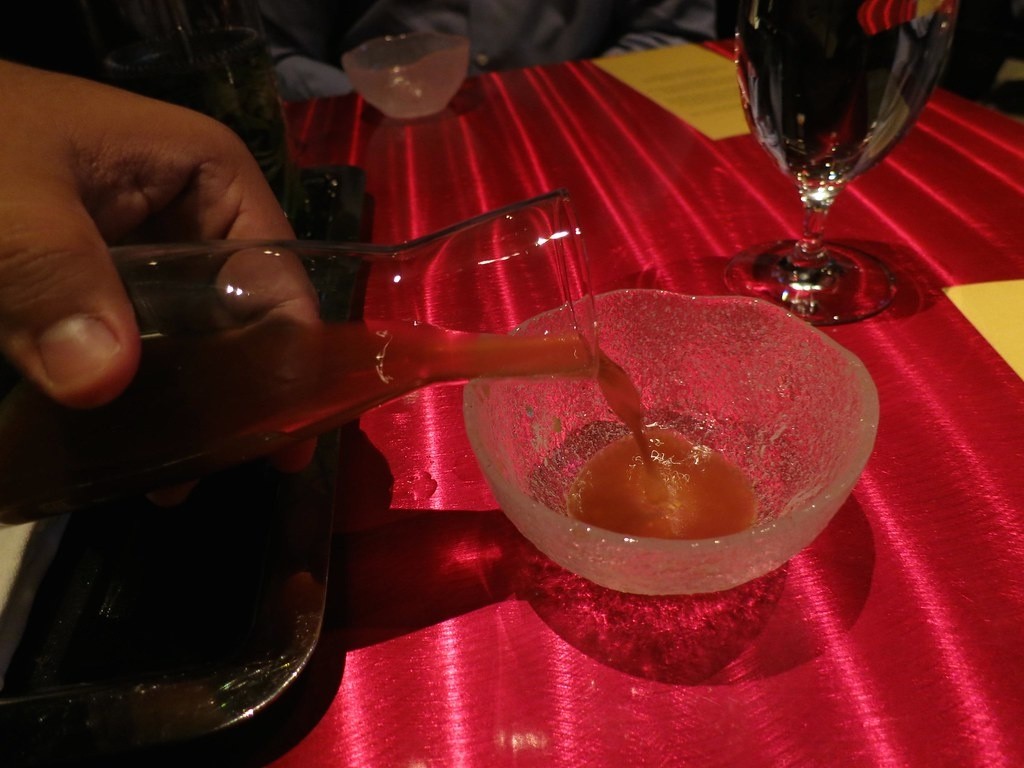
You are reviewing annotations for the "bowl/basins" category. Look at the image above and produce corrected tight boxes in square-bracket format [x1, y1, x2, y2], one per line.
[463, 291, 878, 594]
[342, 32, 469, 118]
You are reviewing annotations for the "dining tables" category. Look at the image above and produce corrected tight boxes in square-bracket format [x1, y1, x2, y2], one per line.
[2, 38, 1024, 767]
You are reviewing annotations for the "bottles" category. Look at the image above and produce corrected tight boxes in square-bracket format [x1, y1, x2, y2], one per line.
[0, 189, 599, 525]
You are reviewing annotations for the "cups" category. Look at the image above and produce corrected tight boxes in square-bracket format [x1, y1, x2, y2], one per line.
[84, 0, 287, 181]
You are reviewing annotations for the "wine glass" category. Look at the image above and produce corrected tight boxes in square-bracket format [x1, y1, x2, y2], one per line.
[723, 0, 956, 324]
